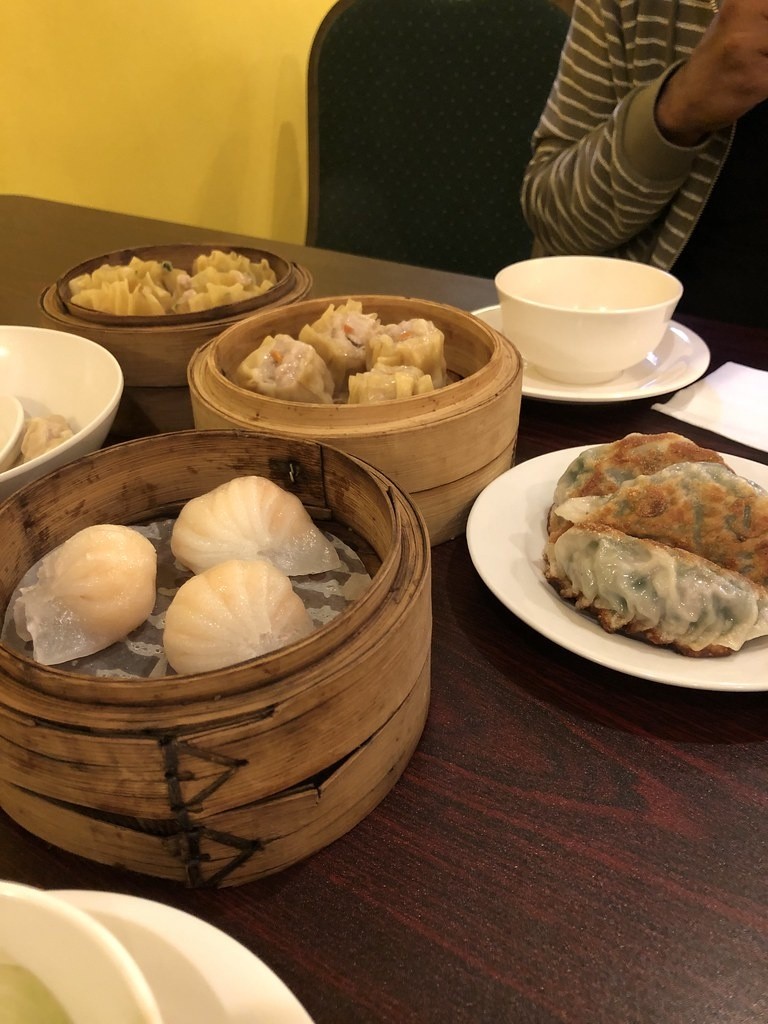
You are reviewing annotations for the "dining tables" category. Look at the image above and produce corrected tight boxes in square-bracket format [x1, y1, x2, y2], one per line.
[0, 192, 768, 1024]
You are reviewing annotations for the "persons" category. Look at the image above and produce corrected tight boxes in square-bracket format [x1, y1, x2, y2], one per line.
[520, 0, 768, 332]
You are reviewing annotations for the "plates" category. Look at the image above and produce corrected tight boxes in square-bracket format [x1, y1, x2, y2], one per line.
[47, 891, 313, 1024]
[471, 304, 710, 402]
[467, 444, 768, 692]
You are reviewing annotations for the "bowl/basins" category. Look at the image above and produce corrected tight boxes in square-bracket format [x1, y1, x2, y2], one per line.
[494, 254, 683, 385]
[1, 324, 124, 501]
[0, 880, 163, 1024]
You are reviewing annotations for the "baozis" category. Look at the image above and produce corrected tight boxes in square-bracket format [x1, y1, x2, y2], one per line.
[12, 473, 341, 677]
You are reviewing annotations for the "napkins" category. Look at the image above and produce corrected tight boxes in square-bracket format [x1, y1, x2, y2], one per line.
[651, 359, 767, 453]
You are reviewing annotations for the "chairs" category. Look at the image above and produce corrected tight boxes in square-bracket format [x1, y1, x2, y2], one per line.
[304, 0, 575, 280]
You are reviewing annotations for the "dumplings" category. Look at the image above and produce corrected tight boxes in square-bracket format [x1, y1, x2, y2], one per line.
[541, 431, 768, 657]
